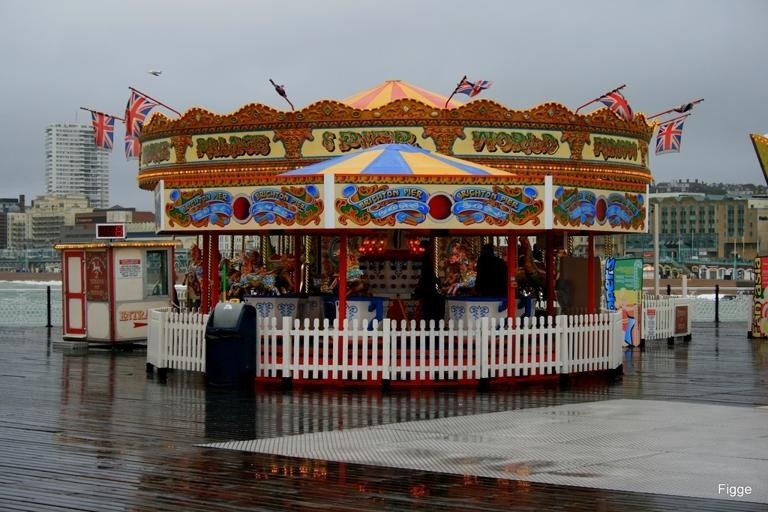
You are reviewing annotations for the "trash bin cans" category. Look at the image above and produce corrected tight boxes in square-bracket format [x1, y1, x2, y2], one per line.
[204, 303, 256, 402]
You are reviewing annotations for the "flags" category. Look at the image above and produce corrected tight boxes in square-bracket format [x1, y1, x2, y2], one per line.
[125, 91, 157, 138]
[656, 118, 687, 153]
[91, 112, 113, 149]
[454, 80, 494, 97]
[125, 123, 144, 159]
[599, 89, 634, 122]
